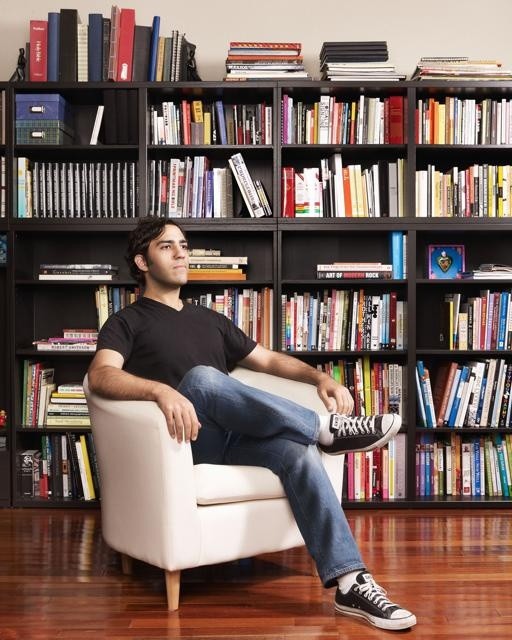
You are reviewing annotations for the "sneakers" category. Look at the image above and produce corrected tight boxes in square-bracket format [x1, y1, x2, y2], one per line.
[314, 407, 403, 460]
[330, 569, 418, 632]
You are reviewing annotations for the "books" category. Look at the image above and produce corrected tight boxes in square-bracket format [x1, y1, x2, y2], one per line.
[18, 157, 139, 219]
[415, 97, 512, 218]
[223, 41, 512, 81]
[312, 356, 407, 501]
[430, 264, 512, 351]
[184, 249, 274, 351]
[416, 359, 512, 496]
[282, 232, 408, 352]
[1, 89, 7, 501]
[281, 94, 408, 218]
[90, 89, 138, 145]
[26, 5, 196, 82]
[149, 100, 274, 219]
[16, 264, 143, 501]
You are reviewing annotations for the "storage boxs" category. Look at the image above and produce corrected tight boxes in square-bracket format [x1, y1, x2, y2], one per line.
[14, 92, 75, 146]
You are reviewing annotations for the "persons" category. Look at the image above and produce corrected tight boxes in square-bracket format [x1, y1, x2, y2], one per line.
[17, 48, 27, 81]
[86, 215, 420, 633]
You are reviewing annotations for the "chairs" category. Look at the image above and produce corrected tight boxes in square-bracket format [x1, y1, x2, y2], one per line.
[82, 351, 352, 612]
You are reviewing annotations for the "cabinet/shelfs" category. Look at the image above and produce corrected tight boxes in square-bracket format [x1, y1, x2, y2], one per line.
[2, 79, 512, 507]
